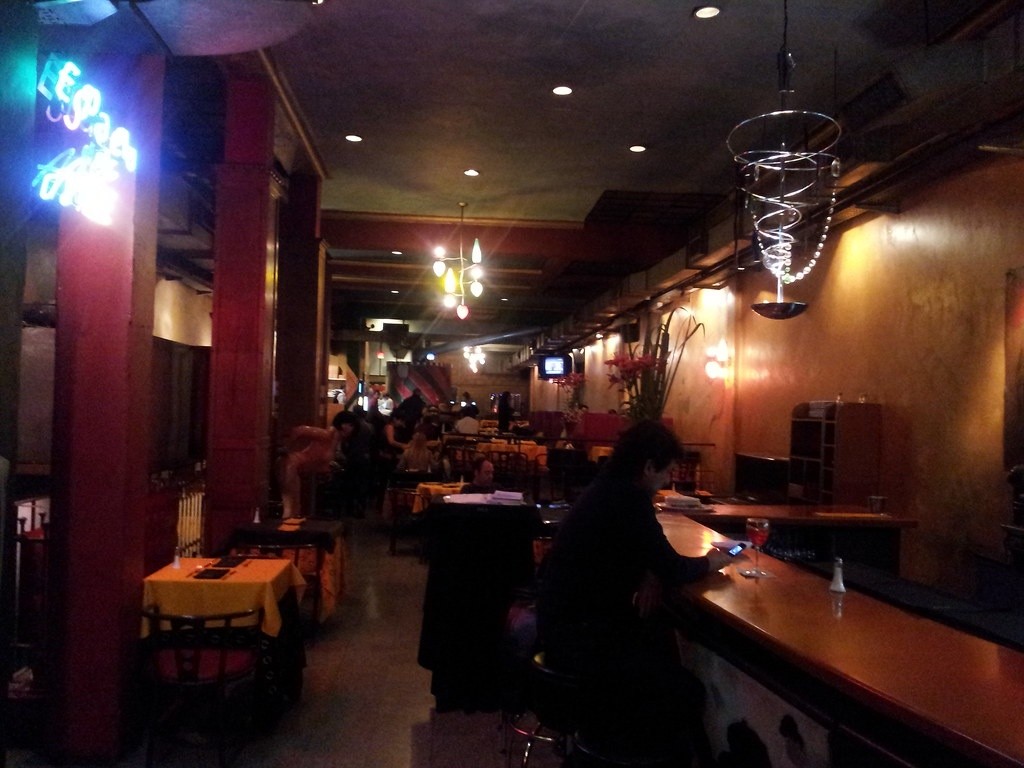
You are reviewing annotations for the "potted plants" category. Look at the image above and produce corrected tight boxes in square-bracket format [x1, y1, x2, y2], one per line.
[674, 451, 702, 491]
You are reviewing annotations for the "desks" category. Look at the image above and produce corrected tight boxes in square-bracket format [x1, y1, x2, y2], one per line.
[235, 518, 344, 622]
[412, 481, 471, 564]
[141, 558, 308, 700]
[424, 439, 537, 474]
[442, 492, 565, 537]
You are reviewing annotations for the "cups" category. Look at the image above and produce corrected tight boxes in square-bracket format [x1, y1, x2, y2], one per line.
[773, 547, 817, 561]
[869, 496, 888, 513]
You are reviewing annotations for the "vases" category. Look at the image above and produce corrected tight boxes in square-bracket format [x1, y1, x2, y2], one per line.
[563, 421, 578, 449]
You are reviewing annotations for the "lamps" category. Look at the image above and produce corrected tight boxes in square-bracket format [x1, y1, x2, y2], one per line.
[433, 204, 486, 318]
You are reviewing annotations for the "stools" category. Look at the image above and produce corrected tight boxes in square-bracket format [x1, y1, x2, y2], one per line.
[497, 602, 681, 768]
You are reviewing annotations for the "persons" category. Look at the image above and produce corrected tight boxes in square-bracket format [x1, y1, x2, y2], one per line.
[535, 421, 734, 768]
[318, 388, 534, 516]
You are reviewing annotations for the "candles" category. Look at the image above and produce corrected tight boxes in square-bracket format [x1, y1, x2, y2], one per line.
[175, 491, 202, 557]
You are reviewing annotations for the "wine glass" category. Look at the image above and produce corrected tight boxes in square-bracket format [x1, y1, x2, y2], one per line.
[745, 518, 769, 575]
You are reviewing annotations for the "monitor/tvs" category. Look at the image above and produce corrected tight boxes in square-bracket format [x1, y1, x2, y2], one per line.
[540, 355, 572, 380]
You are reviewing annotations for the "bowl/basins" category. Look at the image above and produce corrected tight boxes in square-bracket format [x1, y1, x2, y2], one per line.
[710, 541, 753, 552]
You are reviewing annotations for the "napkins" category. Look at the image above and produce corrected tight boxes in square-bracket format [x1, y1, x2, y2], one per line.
[493, 490, 523, 500]
[282, 518, 306, 524]
[213, 558, 247, 568]
[194, 569, 228, 579]
[278, 524, 300, 531]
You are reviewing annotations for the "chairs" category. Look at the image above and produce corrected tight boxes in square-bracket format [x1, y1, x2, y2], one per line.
[223, 544, 322, 638]
[388, 472, 439, 565]
[137, 604, 264, 768]
[448, 447, 528, 479]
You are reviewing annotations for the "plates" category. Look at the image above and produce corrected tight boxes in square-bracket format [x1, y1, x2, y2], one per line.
[656, 502, 713, 511]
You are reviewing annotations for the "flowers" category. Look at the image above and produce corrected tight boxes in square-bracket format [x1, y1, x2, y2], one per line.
[603, 303, 705, 416]
[554, 372, 593, 422]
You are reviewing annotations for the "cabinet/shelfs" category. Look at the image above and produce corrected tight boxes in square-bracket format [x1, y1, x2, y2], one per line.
[788, 401, 881, 504]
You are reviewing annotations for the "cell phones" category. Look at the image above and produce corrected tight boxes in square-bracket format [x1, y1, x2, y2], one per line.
[727, 542, 747, 557]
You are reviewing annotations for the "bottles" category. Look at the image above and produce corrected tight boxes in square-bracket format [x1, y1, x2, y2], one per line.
[172, 547, 181, 569]
[829, 557, 846, 593]
[252, 507, 261, 523]
[149, 460, 207, 493]
